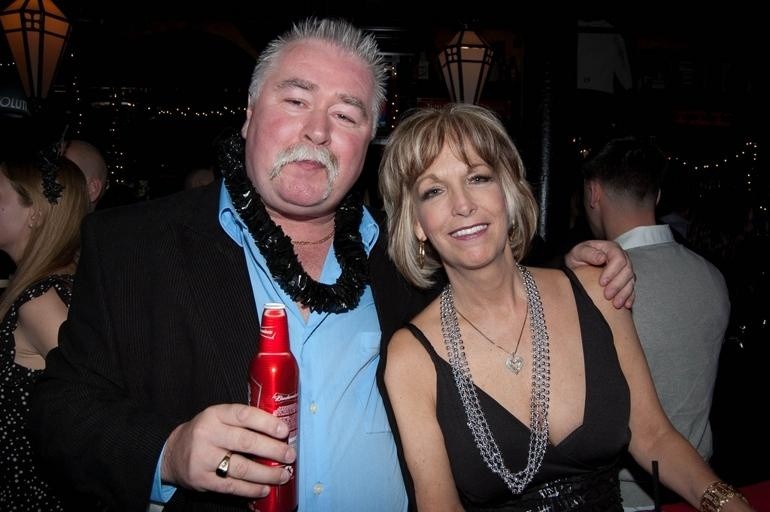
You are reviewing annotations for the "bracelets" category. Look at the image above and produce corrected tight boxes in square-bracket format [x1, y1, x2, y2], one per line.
[699, 481, 752, 512]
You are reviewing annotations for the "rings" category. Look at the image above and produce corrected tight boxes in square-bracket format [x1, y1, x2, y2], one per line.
[216, 452, 233, 477]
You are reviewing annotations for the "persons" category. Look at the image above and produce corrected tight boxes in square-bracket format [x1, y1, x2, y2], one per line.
[184, 166, 213, 189]
[55, 138, 113, 257]
[28, 20, 637, 512]
[582, 133, 732, 499]
[0, 143, 92, 512]
[379, 103, 753, 512]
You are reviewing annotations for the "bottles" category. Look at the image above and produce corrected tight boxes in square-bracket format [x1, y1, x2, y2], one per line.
[243, 299, 301, 512]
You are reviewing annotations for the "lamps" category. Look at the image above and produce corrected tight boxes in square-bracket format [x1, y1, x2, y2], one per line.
[436, 20, 495, 107]
[0, 0, 73, 100]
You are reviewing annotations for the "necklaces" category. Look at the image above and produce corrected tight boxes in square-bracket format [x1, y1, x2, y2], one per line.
[440, 262, 553, 496]
[454, 304, 530, 376]
